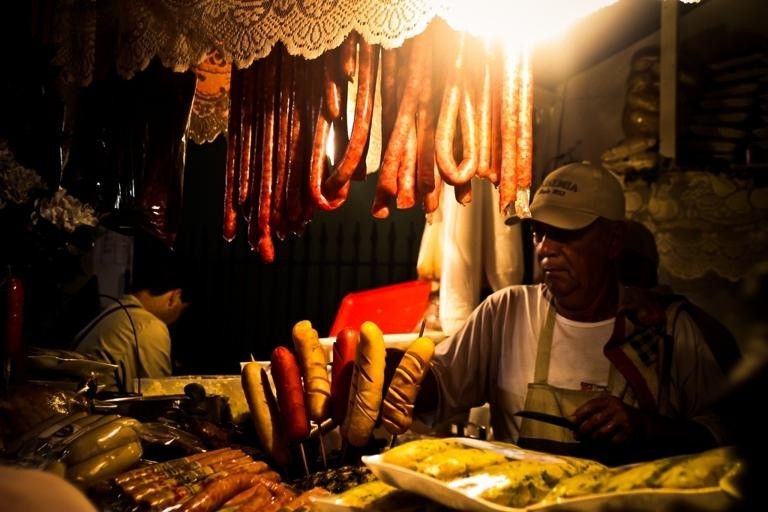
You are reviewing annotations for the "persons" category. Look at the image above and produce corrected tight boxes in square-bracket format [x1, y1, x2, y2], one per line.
[68, 258, 197, 380]
[377, 159, 732, 468]
[617, 218, 741, 375]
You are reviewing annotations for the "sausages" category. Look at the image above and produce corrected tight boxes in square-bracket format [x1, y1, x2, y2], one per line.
[3, 277, 24, 355]
[7, 318, 436, 512]
[221, 14, 534, 262]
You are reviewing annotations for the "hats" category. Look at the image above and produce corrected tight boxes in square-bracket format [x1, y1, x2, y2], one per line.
[504, 162, 627, 232]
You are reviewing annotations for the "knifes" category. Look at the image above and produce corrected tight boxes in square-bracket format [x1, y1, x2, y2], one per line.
[510, 406, 580, 431]
